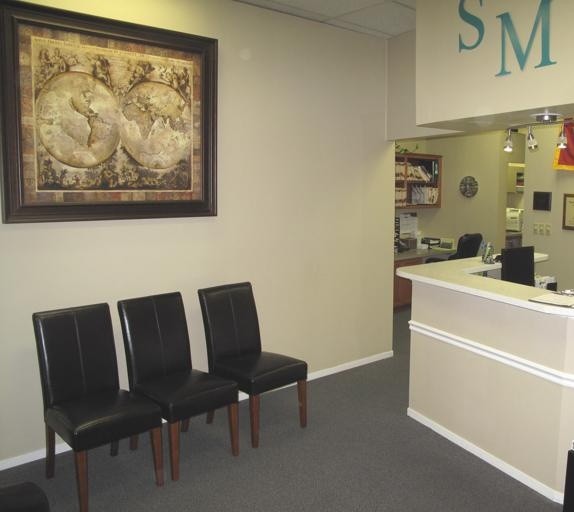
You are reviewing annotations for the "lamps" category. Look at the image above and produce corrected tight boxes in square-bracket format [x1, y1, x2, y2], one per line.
[531, 113, 560, 125]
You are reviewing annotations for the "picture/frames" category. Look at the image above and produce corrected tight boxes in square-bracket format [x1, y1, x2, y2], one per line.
[562, 193, 574, 229]
[0, 0, 218, 224]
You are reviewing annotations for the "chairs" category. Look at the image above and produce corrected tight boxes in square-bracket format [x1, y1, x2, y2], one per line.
[500, 121, 571, 152]
[423, 233, 483, 264]
[33, 302, 165, 511]
[118, 292, 240, 481]
[0, 482, 50, 512]
[198, 282, 309, 448]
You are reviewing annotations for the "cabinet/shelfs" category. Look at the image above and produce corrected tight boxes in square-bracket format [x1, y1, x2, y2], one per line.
[506, 164, 524, 194]
[396, 152, 442, 208]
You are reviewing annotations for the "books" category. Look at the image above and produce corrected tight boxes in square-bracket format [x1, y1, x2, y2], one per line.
[421, 236, 457, 254]
[395, 157, 440, 208]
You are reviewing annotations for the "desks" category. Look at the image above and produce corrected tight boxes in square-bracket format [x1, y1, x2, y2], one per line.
[394, 250, 458, 313]
